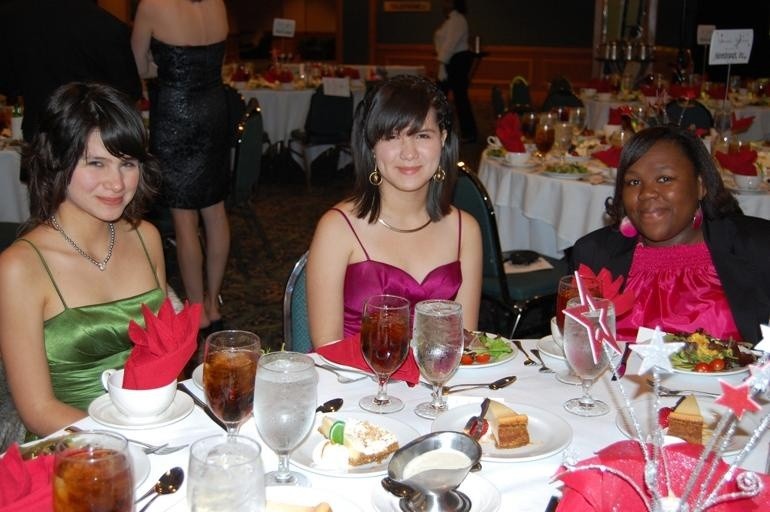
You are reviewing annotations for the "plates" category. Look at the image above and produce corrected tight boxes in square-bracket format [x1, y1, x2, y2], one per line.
[286, 410, 423, 478]
[89, 391, 195, 432]
[432, 401, 573, 461]
[616, 394, 761, 456]
[127, 444, 151, 492]
[536, 334, 566, 360]
[636, 336, 759, 377]
[409, 330, 521, 369]
[192, 360, 204, 393]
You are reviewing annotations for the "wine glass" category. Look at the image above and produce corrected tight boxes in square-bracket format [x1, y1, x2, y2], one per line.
[253, 350, 318, 487]
[380, 429, 483, 511]
[563, 295, 617, 419]
[556, 275, 604, 386]
[411, 299, 464, 420]
[358, 295, 411, 414]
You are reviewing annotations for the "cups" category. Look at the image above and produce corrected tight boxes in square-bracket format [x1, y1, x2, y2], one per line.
[580, 38, 766, 102]
[202, 329, 262, 436]
[187, 437, 267, 512]
[52, 429, 135, 512]
[486, 104, 760, 192]
[277, 51, 311, 86]
[551, 316, 569, 351]
[102, 367, 180, 420]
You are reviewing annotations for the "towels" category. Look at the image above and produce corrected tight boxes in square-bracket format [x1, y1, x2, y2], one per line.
[310, 332, 420, 389]
[0, 440, 69, 510]
[110, 292, 205, 390]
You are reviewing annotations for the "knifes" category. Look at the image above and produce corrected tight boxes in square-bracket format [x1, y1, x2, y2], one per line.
[178, 383, 229, 433]
[612, 341, 636, 382]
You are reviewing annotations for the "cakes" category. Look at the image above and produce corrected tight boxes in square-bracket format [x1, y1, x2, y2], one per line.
[345, 415, 399, 472]
[270, 502, 333, 512]
[484, 399, 530, 450]
[669, 392, 704, 444]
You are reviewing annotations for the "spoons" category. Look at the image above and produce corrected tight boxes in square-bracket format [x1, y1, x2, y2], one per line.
[139, 467, 184, 512]
[443, 376, 517, 396]
[316, 398, 343, 414]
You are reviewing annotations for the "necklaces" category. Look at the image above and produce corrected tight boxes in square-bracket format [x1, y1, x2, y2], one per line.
[47, 214, 124, 272]
[370, 207, 441, 233]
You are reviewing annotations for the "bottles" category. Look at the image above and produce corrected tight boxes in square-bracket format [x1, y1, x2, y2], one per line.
[11, 102, 22, 118]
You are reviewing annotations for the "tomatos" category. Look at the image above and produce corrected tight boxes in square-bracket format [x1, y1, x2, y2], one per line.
[694, 362, 709, 373]
[461, 354, 474, 364]
[711, 359, 725, 371]
[476, 354, 490, 363]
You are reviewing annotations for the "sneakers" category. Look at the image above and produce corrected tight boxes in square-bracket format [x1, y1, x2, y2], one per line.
[198, 316, 224, 338]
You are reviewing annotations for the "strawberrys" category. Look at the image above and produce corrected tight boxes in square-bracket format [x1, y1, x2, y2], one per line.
[658, 407, 673, 427]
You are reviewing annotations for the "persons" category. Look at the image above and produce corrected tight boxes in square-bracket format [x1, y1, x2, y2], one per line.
[431, 1, 484, 138]
[305, 71, 478, 351]
[2, 81, 185, 442]
[0, 0, 145, 207]
[129, 2, 249, 329]
[555, 134, 770, 354]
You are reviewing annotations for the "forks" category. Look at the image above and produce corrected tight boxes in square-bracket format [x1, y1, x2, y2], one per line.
[63, 426, 188, 455]
[513, 340, 553, 374]
[312, 359, 401, 384]
